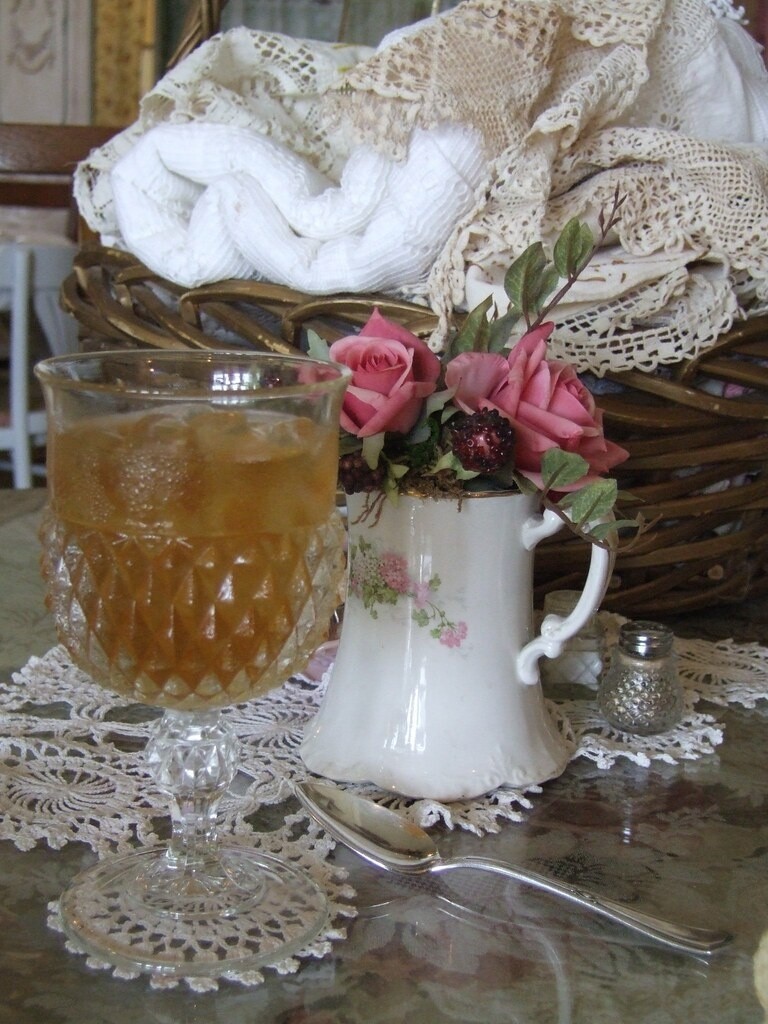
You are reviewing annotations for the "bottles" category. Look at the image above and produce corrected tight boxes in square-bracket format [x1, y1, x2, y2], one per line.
[535, 588, 605, 699]
[600, 622, 683, 734]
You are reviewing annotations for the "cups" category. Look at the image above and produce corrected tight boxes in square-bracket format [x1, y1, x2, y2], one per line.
[295, 488, 618, 799]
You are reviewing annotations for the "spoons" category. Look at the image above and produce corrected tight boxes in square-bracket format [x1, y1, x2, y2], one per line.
[297, 783, 732, 955]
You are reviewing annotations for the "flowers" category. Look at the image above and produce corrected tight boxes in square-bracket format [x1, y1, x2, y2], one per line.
[253, 178, 667, 553]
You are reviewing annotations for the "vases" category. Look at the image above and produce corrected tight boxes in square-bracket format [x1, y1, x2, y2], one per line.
[300, 482, 617, 799]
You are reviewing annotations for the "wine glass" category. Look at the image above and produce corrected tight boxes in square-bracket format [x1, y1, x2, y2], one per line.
[35, 349, 354, 975]
